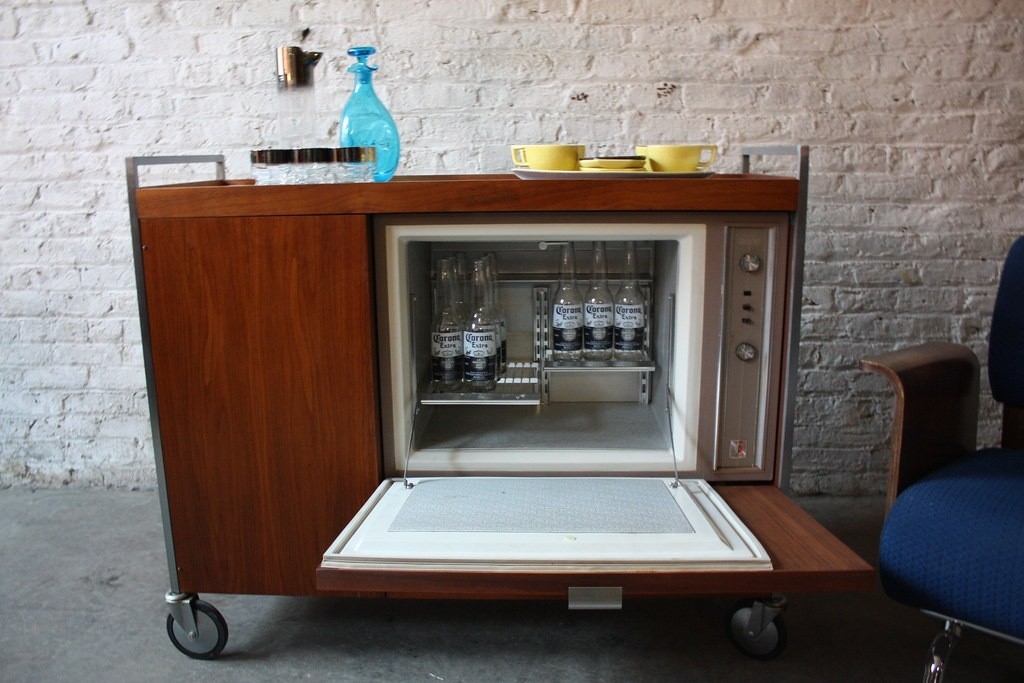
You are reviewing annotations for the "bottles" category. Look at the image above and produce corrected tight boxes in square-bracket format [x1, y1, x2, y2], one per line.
[340, 47, 399, 182]
[430, 252, 506, 395]
[585, 241, 614, 361]
[615, 241, 645, 361]
[553, 241, 583, 361]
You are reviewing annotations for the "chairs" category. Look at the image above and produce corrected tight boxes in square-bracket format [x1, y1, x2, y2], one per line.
[860, 235, 1024, 683]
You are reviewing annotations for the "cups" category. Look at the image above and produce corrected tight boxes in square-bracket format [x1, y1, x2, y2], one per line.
[250, 149, 291, 185]
[337, 148, 375, 184]
[636, 145, 717, 172]
[292, 148, 336, 186]
[510, 144, 584, 172]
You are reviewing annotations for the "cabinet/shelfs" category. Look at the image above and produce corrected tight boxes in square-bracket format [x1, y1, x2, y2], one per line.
[126, 144, 877, 662]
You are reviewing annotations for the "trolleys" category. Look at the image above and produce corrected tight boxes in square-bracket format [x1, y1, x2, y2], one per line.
[124, 154, 877, 659]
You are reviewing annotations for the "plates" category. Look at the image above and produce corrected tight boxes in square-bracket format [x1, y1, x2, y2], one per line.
[579, 156, 646, 173]
